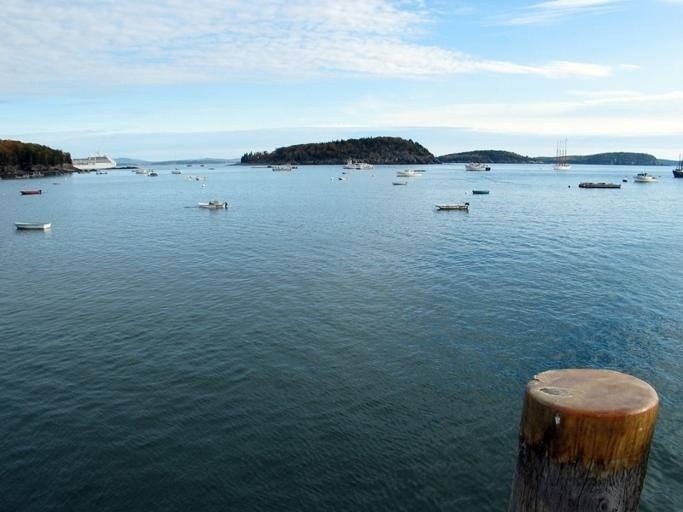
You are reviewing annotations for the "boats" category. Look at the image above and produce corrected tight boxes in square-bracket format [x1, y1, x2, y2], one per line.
[132, 167, 159, 176]
[434, 202, 469, 209]
[198, 201, 228, 208]
[343, 159, 373, 170]
[672, 153, 683, 178]
[252, 165, 297, 171]
[578, 181, 621, 189]
[96, 172, 107, 175]
[466, 163, 491, 171]
[473, 189, 490, 194]
[392, 168, 425, 185]
[15, 222, 51, 229]
[20, 189, 41, 194]
[171, 170, 206, 181]
[633, 172, 660, 182]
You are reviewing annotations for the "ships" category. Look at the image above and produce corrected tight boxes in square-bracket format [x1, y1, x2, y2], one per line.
[72, 155, 117, 171]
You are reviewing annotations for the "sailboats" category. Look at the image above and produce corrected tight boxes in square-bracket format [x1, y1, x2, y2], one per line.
[553, 136, 570, 170]
